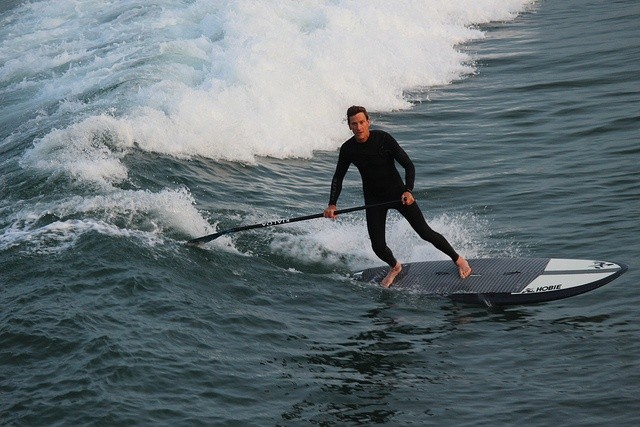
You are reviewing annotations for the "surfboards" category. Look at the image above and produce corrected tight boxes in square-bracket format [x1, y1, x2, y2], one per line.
[357, 257, 627, 307]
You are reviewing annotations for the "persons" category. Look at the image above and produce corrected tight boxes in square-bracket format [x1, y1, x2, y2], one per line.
[323, 105, 472, 288]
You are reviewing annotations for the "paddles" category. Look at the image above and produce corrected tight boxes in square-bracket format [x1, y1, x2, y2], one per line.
[189, 197, 408, 242]
